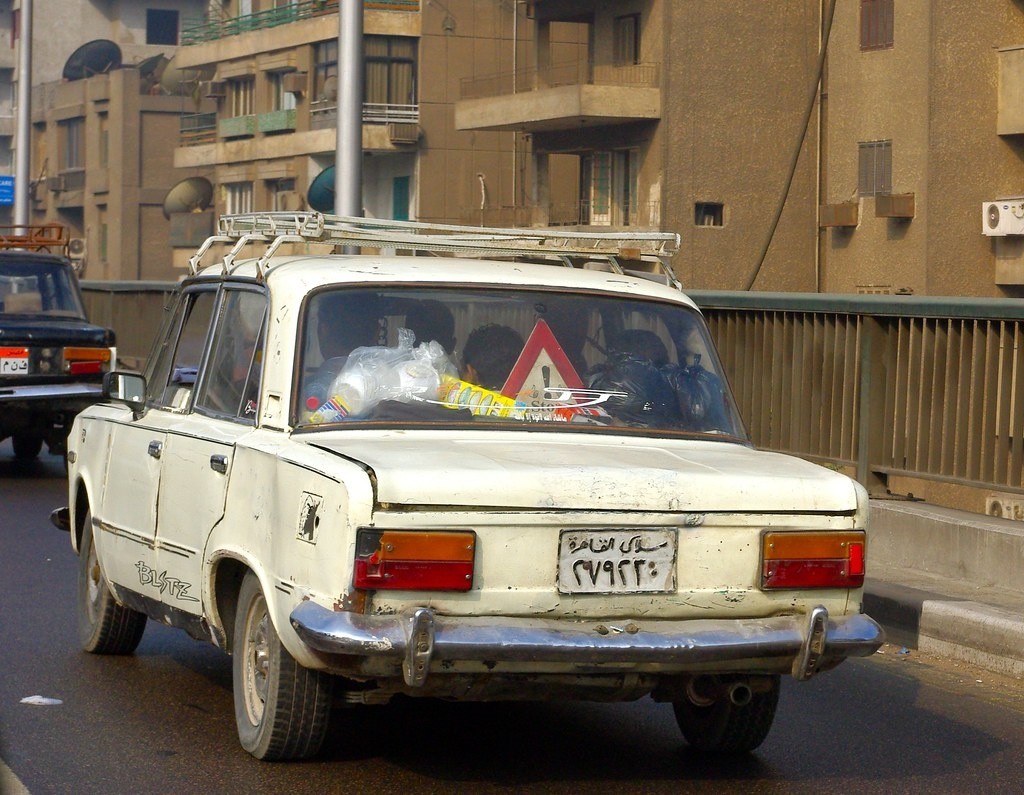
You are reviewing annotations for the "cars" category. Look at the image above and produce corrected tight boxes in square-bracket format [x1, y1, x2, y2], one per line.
[49, 211, 886, 761]
[0, 223, 116, 460]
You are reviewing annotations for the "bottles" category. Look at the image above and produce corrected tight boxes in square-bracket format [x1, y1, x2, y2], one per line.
[304, 372, 338, 410]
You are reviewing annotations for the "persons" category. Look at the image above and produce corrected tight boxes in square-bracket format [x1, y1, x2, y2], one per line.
[460, 324, 525, 391]
[405, 300, 457, 355]
[317, 287, 389, 361]
[605, 330, 670, 373]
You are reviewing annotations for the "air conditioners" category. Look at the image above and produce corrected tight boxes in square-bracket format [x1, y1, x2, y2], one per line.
[65, 238, 87, 259]
[980, 200, 1024, 237]
[277, 191, 304, 211]
